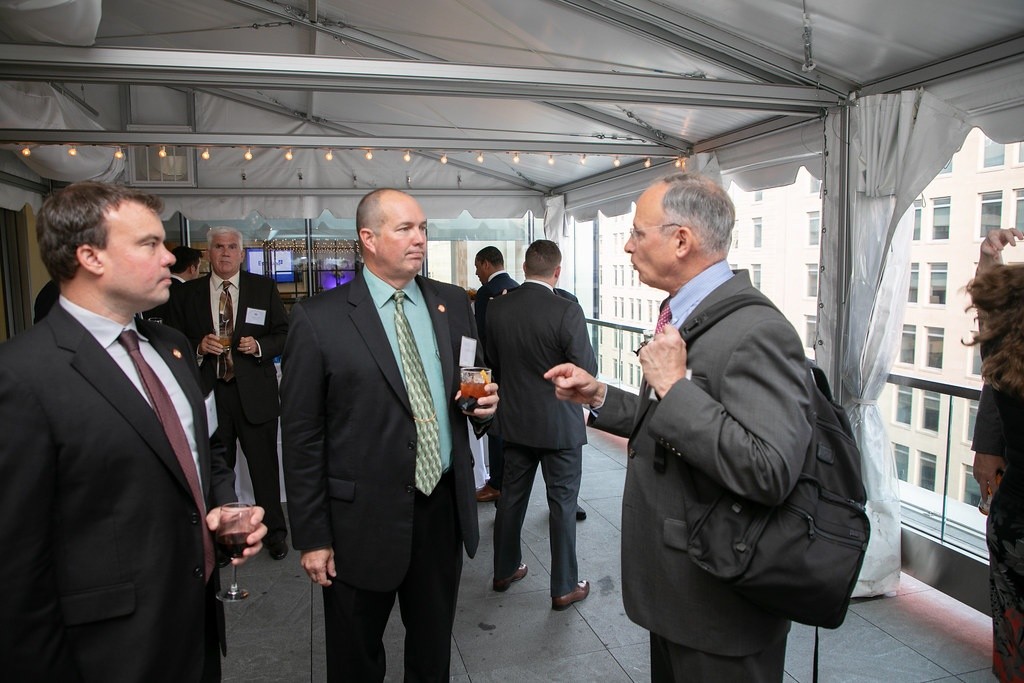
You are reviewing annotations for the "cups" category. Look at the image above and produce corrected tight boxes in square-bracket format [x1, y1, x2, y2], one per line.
[459, 367, 491, 417]
[215, 328, 232, 351]
[150, 318, 164, 324]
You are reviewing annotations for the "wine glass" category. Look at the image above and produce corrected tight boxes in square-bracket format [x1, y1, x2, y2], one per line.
[214, 503, 251, 601]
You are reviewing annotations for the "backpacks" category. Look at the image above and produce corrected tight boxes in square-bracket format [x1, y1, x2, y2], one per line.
[677, 294, 872, 631]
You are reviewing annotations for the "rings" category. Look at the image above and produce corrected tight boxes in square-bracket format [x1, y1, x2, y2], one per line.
[248, 346, 250, 350]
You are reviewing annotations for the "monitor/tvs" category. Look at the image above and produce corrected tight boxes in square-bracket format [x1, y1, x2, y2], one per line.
[247, 249, 295, 283]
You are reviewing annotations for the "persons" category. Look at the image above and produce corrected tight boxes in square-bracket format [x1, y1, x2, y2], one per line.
[466, 239, 598, 612]
[34, 280, 52, 325]
[0, 180, 269, 683]
[166, 225, 289, 568]
[280, 188, 499, 683]
[142, 245, 204, 326]
[544, 173, 806, 682]
[966, 228, 1024, 683]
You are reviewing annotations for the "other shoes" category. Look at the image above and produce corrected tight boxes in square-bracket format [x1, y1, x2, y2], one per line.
[215, 551, 231, 568]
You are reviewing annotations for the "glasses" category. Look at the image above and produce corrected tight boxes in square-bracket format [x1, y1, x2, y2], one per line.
[629, 223, 683, 239]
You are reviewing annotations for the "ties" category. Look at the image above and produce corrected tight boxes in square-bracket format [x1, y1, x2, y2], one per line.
[655, 298, 672, 340]
[392, 288, 443, 496]
[117, 329, 216, 587]
[218, 279, 236, 383]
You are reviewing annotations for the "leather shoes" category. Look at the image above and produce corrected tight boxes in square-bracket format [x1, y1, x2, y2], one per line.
[492, 562, 528, 592]
[478, 483, 500, 501]
[552, 579, 590, 612]
[269, 540, 287, 561]
[576, 504, 586, 520]
